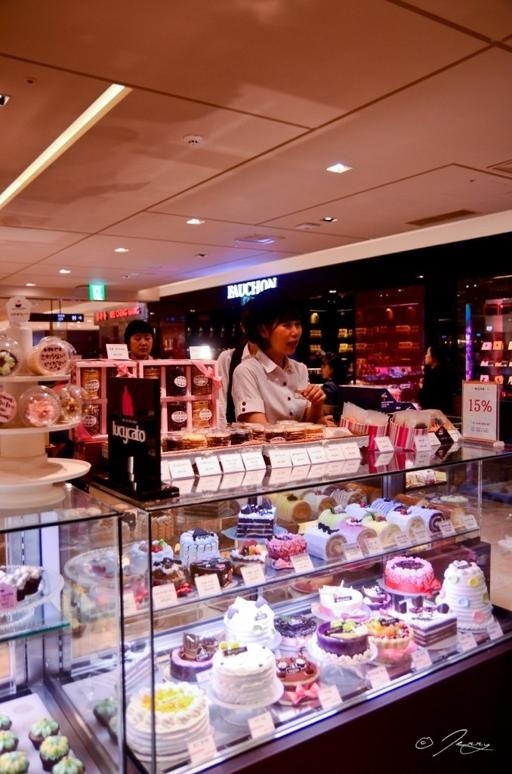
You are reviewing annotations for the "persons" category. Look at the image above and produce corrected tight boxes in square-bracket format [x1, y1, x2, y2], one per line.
[414, 341, 460, 415]
[230, 284, 339, 428]
[212, 298, 267, 426]
[122, 318, 157, 360]
[319, 351, 349, 384]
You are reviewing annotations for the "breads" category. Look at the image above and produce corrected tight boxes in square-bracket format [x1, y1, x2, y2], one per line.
[0, 493, 500, 774]
[163, 421, 324, 452]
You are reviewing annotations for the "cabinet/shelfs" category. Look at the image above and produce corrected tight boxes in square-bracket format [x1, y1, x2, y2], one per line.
[1, 423, 511, 774]
[2, 326, 220, 511]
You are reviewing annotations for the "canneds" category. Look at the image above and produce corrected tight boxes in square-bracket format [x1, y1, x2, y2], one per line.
[80, 368, 212, 434]
